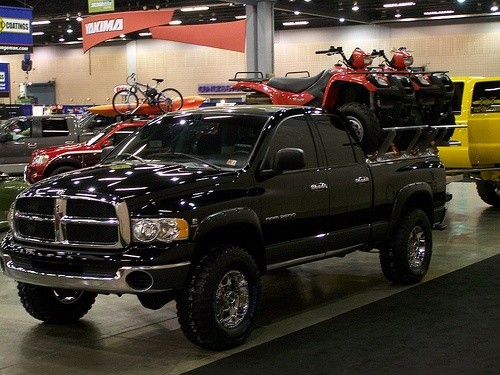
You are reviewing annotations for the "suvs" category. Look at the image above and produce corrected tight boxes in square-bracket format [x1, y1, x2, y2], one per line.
[25, 120, 153, 184]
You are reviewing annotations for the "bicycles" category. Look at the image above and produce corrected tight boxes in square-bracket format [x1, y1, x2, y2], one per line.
[112, 72, 184, 117]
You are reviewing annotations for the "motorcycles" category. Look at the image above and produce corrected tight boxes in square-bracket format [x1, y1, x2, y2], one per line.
[229, 45, 457, 153]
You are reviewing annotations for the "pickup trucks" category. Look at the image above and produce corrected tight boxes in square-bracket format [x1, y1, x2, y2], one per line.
[0, 115, 104, 179]
[0, 103, 455, 351]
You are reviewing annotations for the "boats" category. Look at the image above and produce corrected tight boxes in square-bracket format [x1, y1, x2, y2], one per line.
[87, 96, 207, 116]
